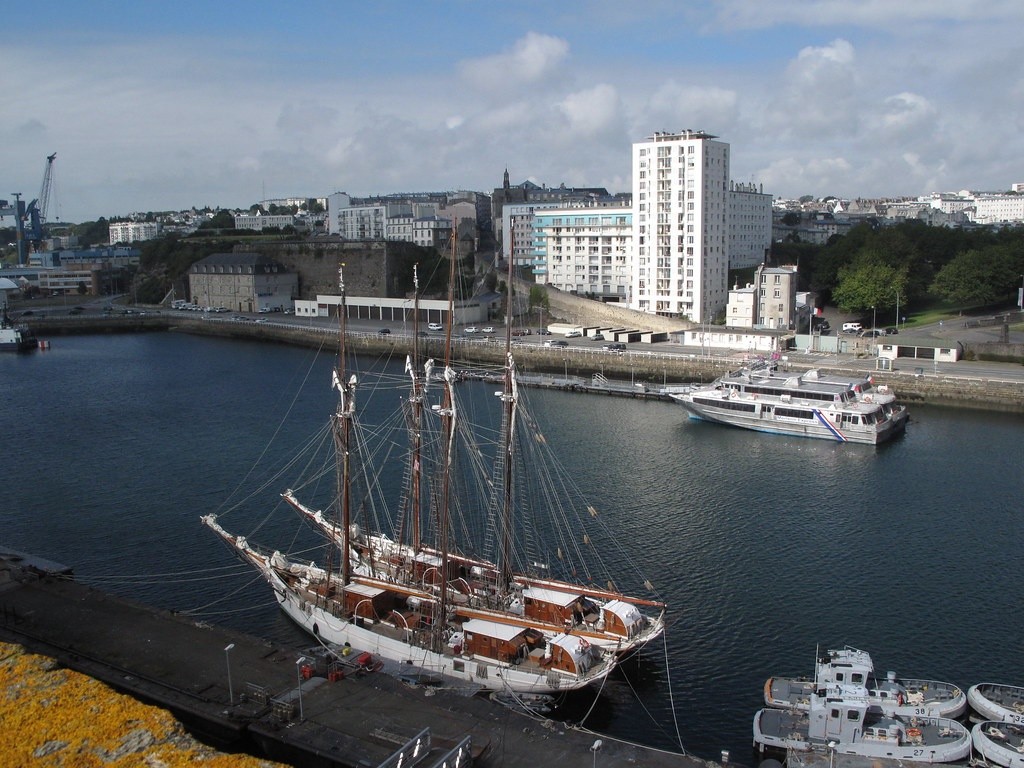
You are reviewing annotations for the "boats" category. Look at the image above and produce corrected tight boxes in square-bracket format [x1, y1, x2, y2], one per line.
[973, 722, 1024, 768]
[665, 365, 911, 444]
[754, 684, 971, 762]
[762, 644, 965, 718]
[0, 319, 35, 353]
[967, 682, 1024, 724]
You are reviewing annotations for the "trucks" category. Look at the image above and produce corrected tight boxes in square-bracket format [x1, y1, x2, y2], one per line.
[843, 323, 862, 332]
[199, 214, 666, 694]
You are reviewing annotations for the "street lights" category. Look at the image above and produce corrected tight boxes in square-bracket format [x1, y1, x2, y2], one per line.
[809, 313, 814, 356]
[871, 306, 876, 357]
[891, 286, 900, 329]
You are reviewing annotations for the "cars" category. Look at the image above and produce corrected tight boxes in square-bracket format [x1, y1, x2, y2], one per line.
[231, 315, 267, 323]
[512, 329, 531, 336]
[603, 344, 626, 351]
[378, 329, 390, 334]
[284, 309, 295, 315]
[140, 311, 147, 315]
[818, 320, 829, 329]
[413, 331, 429, 337]
[536, 329, 551, 335]
[857, 327, 899, 337]
[188, 305, 227, 313]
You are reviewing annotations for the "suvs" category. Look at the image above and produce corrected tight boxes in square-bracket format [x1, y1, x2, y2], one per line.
[463, 327, 479, 333]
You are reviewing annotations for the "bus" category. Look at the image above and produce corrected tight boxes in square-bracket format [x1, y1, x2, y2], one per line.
[171, 300, 186, 308]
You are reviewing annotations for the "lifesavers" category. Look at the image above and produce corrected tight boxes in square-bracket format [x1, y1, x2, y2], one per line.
[907, 728, 921, 736]
[579, 638, 590, 649]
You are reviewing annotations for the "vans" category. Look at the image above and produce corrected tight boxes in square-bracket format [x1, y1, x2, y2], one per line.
[481, 327, 496, 333]
[179, 303, 192, 310]
[545, 340, 558, 346]
[258, 308, 270, 314]
[590, 334, 603, 341]
[565, 331, 581, 338]
[428, 323, 442, 331]
